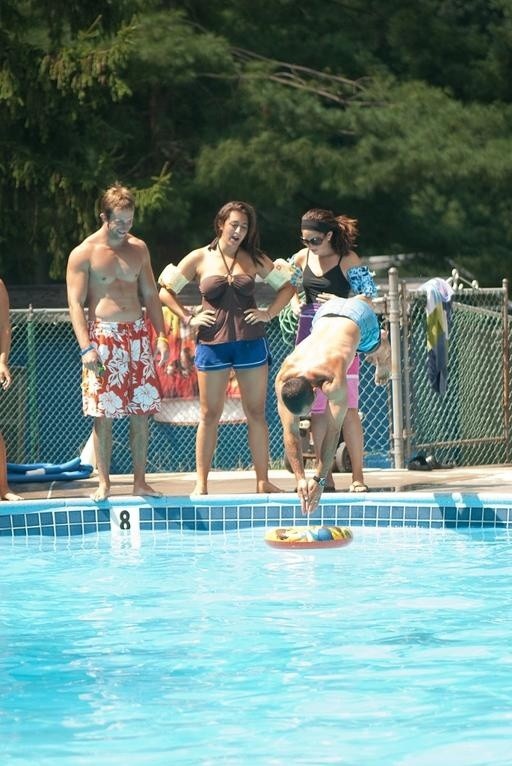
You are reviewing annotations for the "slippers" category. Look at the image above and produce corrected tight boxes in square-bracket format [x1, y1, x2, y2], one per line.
[294, 484, 368, 492]
[407, 455, 453, 471]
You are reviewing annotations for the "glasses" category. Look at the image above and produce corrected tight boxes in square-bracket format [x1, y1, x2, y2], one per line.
[300, 234, 327, 246]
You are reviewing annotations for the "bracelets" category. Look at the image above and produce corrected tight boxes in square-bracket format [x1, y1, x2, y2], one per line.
[263, 310, 273, 323]
[154, 336, 170, 344]
[77, 345, 96, 357]
[180, 312, 194, 325]
[312, 474, 329, 487]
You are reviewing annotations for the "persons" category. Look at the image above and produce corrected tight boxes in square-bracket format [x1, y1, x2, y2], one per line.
[274, 297, 393, 515]
[1, 280, 26, 503]
[288, 207, 376, 494]
[65, 186, 172, 502]
[159, 200, 295, 495]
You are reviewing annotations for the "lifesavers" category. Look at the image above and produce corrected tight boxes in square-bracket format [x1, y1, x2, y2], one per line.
[265, 525, 352, 549]
